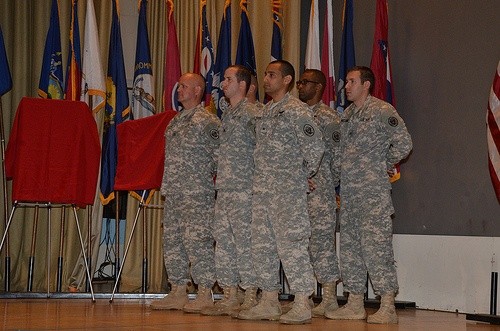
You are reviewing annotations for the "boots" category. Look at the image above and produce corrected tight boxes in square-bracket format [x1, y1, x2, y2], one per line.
[325, 293, 367, 320]
[311, 280, 340, 317]
[279, 293, 311, 324]
[228, 289, 260, 318]
[237, 290, 282, 320]
[150, 285, 189, 308]
[200, 286, 240, 315]
[183, 288, 213, 313]
[367, 295, 398, 324]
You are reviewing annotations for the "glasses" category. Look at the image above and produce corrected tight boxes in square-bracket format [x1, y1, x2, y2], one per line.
[296, 79, 319, 85]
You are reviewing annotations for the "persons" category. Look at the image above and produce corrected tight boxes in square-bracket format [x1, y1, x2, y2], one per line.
[149, 73, 221, 312]
[280, 69, 343, 317]
[238, 59, 324, 324]
[203, 64, 259, 318]
[324, 66, 412, 324]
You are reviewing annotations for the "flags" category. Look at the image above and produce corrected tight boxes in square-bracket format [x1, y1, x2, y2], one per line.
[80, 0, 106, 113]
[235, 0, 260, 102]
[369, 0, 396, 110]
[193, 0, 214, 107]
[38, 0, 64, 100]
[63, 0, 81, 102]
[161, 0, 184, 112]
[263, 0, 284, 105]
[485, 60, 500, 206]
[130, 0, 155, 120]
[211, 0, 232, 118]
[0, 30, 13, 98]
[321, 0, 356, 114]
[304, 0, 321, 71]
[97, 0, 130, 205]
